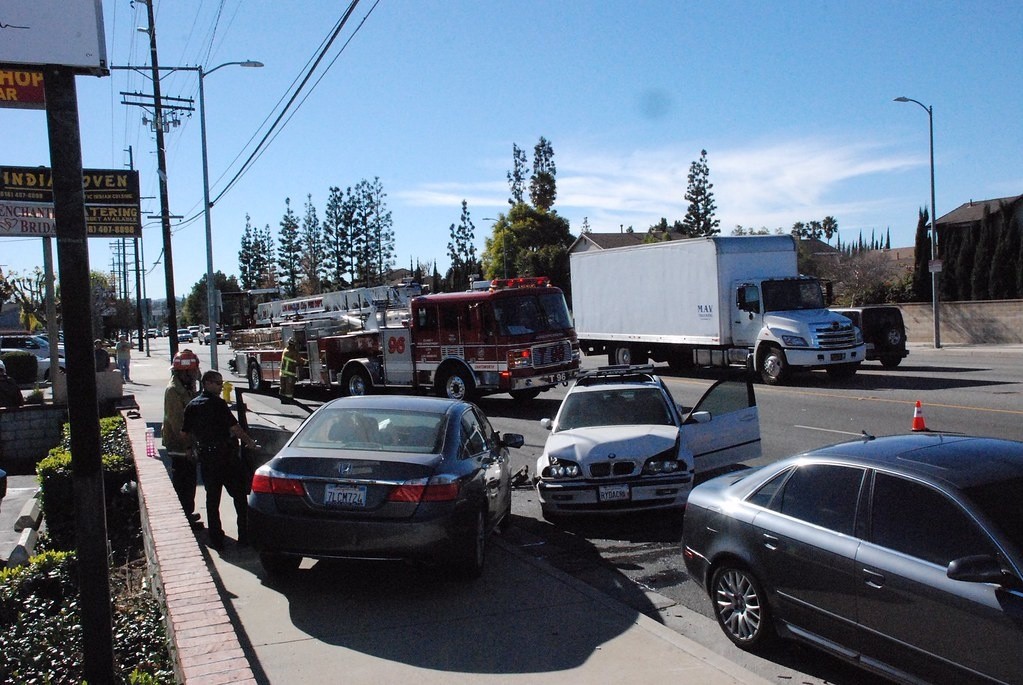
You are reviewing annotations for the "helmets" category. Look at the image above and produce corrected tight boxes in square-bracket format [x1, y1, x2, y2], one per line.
[169, 349, 199, 371]
[287, 337, 298, 346]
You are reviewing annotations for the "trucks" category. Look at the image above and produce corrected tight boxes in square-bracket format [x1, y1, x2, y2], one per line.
[566, 235, 866, 387]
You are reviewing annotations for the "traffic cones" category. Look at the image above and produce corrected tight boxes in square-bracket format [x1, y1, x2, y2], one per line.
[908, 399, 929, 432]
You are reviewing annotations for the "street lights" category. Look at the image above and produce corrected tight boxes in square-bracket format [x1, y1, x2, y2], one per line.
[893, 95, 940, 347]
[195, 60, 265, 372]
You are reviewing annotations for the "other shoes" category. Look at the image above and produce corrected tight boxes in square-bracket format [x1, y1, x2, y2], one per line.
[280, 399, 296, 405]
[185, 512, 204, 528]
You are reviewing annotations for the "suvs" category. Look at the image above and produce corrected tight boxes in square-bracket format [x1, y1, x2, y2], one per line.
[829, 305, 909, 367]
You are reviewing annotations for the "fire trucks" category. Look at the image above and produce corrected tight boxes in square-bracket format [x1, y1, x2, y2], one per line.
[231, 278, 582, 405]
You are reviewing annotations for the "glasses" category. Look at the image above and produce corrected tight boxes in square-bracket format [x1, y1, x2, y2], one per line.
[205, 379, 223, 385]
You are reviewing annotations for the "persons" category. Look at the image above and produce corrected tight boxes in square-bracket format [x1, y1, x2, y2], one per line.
[116, 335, 132, 383]
[163, 351, 202, 524]
[181, 370, 256, 538]
[278, 337, 308, 404]
[93, 338, 110, 373]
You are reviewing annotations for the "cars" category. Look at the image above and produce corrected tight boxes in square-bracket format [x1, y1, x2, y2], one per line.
[535, 363, 765, 527]
[233, 384, 526, 582]
[0, 320, 230, 392]
[678, 432, 1022, 685]
